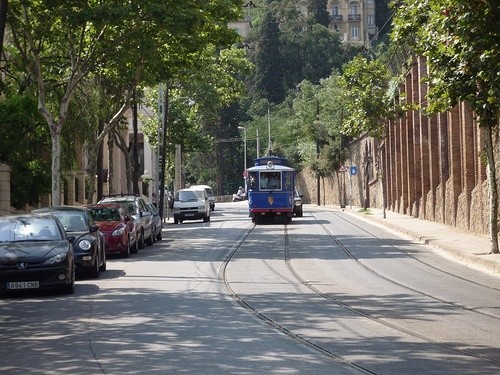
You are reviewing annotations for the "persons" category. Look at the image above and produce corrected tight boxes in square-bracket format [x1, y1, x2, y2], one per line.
[260, 172, 268, 189]
[152, 193, 157, 207]
[232, 186, 246, 201]
[269, 172, 280, 189]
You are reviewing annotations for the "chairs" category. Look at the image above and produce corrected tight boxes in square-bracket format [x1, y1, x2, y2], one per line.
[70, 216, 84, 230]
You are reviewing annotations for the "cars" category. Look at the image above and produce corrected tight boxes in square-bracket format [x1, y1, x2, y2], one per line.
[293, 188, 304, 217]
[81, 204, 139, 259]
[146, 203, 163, 243]
[30, 205, 107, 279]
[0, 213, 77, 296]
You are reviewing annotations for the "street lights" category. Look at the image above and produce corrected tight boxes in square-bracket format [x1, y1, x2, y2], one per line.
[159, 96, 196, 226]
[237, 126, 248, 195]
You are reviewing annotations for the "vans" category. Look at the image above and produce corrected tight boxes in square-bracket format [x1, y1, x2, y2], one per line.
[190, 185, 216, 211]
[173, 188, 211, 225]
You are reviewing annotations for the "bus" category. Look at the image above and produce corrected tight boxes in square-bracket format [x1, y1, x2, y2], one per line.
[245, 148, 296, 224]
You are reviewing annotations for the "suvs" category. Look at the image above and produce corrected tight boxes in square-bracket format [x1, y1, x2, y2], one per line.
[96, 194, 157, 250]
